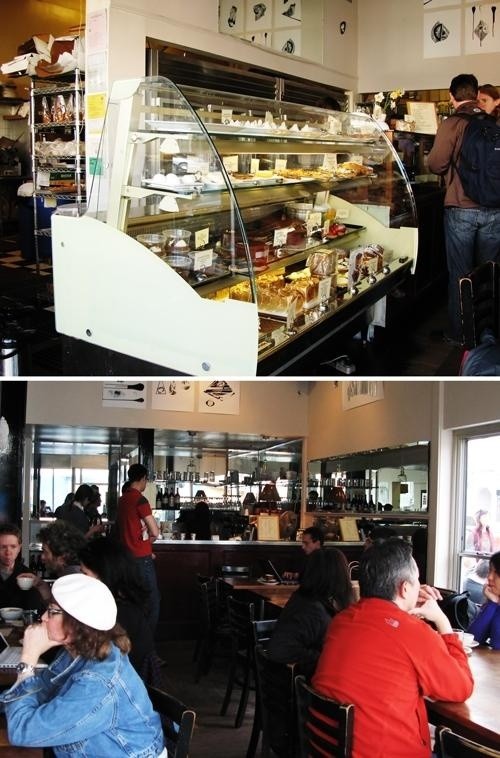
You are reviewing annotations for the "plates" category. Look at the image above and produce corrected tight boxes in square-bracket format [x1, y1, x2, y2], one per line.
[464, 647, 472, 654]
[261, 580, 276, 583]
[468, 641, 480, 647]
[258, 580, 279, 586]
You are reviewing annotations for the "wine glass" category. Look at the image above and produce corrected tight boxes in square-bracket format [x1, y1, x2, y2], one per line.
[181, 495, 242, 512]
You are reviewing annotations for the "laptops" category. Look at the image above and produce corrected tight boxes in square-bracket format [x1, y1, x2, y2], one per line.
[253, 556, 297, 586]
[0, 631, 47, 668]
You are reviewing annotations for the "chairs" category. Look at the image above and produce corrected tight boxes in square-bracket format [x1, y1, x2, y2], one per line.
[435, 726, 500, 757]
[144, 683, 197, 758]
[194, 564, 256, 728]
[245, 620, 279, 757]
[246, 644, 301, 757]
[293, 674, 355, 758]
[458, 259, 500, 376]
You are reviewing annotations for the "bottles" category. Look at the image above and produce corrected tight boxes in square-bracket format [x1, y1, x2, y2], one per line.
[308, 494, 374, 513]
[156, 486, 180, 509]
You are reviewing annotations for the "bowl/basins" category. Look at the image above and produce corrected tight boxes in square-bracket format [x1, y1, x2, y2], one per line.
[135, 228, 218, 284]
[162, 533, 173, 539]
[1, 607, 22, 623]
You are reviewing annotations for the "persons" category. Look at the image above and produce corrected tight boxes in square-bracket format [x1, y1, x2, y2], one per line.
[259, 528, 500, 757]
[384, 504, 394, 511]
[3, 460, 201, 758]
[472, 510, 498, 562]
[424, 71, 500, 360]
[297, 95, 342, 169]
[389, 114, 405, 131]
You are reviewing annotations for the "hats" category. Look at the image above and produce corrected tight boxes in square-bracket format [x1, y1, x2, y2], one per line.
[51, 573, 117, 631]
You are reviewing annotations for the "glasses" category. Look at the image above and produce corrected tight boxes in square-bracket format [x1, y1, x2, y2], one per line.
[48, 608, 63, 618]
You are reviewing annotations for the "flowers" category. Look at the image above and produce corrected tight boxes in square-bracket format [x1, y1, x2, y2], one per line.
[371, 87, 405, 114]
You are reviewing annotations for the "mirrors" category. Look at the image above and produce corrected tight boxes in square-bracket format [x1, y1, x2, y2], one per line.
[304, 440, 432, 517]
[218, 0, 324, 65]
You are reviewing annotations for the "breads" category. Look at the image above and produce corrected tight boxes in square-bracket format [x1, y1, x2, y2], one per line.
[343, 162, 364, 178]
[214, 271, 320, 318]
[310, 243, 384, 293]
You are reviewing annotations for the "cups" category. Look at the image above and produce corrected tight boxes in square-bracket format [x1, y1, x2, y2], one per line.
[264, 575, 273, 580]
[180, 533, 186, 540]
[453, 629, 464, 639]
[191, 533, 197, 541]
[459, 633, 474, 644]
[16, 577, 33, 591]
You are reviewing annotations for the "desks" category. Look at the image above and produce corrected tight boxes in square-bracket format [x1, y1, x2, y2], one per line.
[249, 589, 500, 750]
[0, 619, 44, 758]
[217, 577, 301, 620]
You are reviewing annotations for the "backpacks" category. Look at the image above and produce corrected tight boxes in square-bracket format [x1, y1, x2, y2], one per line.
[449, 112, 500, 207]
[446, 591, 479, 632]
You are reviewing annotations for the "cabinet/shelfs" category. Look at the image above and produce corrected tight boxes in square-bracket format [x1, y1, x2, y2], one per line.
[28, 68, 85, 307]
[152, 479, 300, 512]
[50, 76, 419, 377]
[307, 485, 379, 514]
[385, 130, 447, 328]
